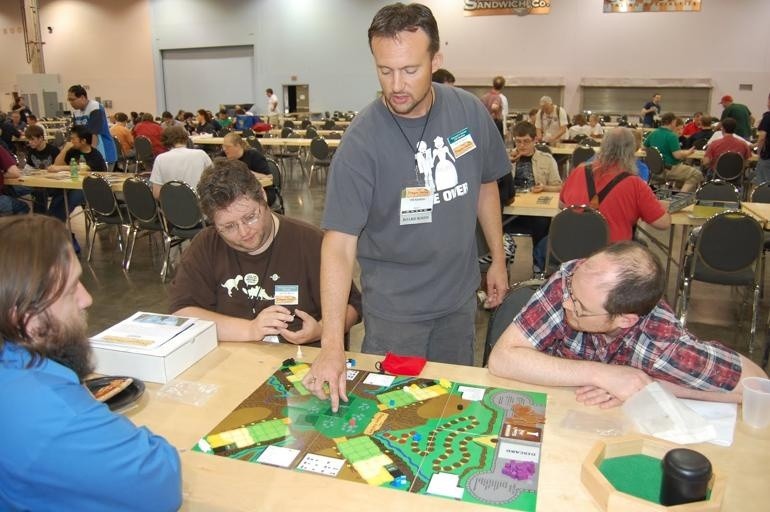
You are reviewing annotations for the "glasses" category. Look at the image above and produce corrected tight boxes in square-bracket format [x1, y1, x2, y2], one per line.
[564, 272, 619, 319]
[213, 198, 262, 234]
[515, 139, 534, 145]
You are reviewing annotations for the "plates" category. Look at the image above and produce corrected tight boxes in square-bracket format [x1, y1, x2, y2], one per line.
[86, 375, 145, 411]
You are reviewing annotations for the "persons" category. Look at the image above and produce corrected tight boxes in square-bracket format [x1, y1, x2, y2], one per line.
[1, 1, 769, 512]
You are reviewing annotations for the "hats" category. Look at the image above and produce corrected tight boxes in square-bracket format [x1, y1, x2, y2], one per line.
[662, 112, 677, 122]
[718, 95, 733, 105]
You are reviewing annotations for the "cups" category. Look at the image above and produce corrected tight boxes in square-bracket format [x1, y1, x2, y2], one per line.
[741, 377, 770, 428]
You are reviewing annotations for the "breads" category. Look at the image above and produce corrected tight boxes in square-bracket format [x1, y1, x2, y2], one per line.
[94, 378, 133, 403]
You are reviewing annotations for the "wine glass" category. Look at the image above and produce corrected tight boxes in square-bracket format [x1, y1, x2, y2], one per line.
[16, 151, 27, 181]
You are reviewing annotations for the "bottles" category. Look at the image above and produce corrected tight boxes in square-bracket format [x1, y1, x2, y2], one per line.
[661, 449, 711, 508]
[69, 158, 77, 180]
[79, 155, 86, 176]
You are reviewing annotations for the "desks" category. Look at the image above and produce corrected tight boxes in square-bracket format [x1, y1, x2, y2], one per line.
[82, 342, 770, 512]
